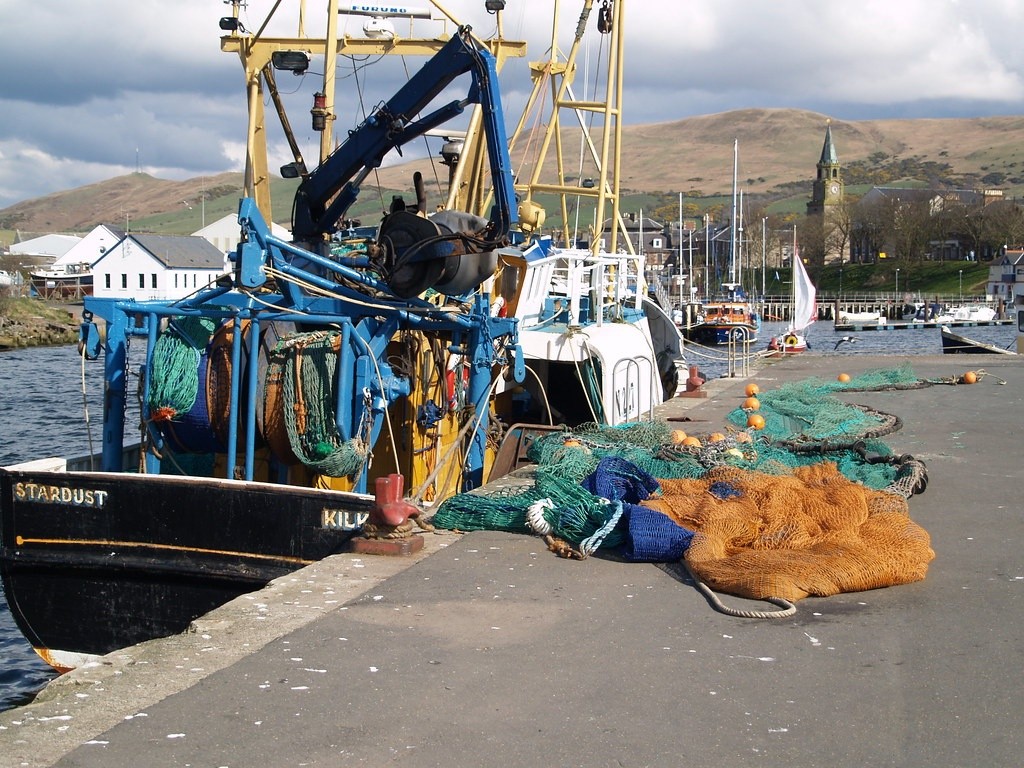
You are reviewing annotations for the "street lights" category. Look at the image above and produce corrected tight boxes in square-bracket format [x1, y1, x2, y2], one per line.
[958, 269, 963, 300]
[895, 268, 900, 301]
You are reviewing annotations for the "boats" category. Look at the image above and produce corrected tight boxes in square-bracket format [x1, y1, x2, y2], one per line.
[0, 0, 1004, 674]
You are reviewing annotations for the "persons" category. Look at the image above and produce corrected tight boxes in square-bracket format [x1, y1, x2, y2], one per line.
[963, 249, 974, 261]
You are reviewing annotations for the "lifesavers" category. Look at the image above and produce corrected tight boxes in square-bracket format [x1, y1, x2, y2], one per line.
[446, 351, 471, 413]
[787, 336, 796, 347]
[489, 296, 508, 351]
